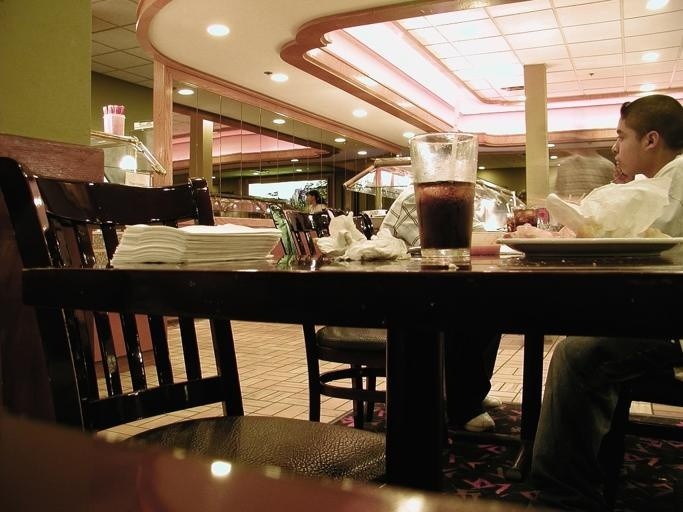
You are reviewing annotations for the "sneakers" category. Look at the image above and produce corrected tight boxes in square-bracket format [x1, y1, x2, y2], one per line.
[537, 489, 603, 509]
[482, 394, 503, 407]
[465, 411, 495, 432]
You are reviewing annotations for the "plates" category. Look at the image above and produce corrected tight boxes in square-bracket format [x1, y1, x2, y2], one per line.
[495, 237, 682, 259]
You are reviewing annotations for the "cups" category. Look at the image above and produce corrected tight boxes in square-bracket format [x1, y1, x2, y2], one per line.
[408, 132, 480, 271]
[512, 208, 537, 230]
[102, 113, 126, 137]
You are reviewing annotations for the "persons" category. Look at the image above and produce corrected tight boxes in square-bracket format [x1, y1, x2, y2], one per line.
[304, 188, 327, 215]
[372, 177, 503, 435]
[527, 90, 681, 512]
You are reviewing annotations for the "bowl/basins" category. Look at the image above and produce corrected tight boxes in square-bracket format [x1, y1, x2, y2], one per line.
[471, 230, 502, 246]
